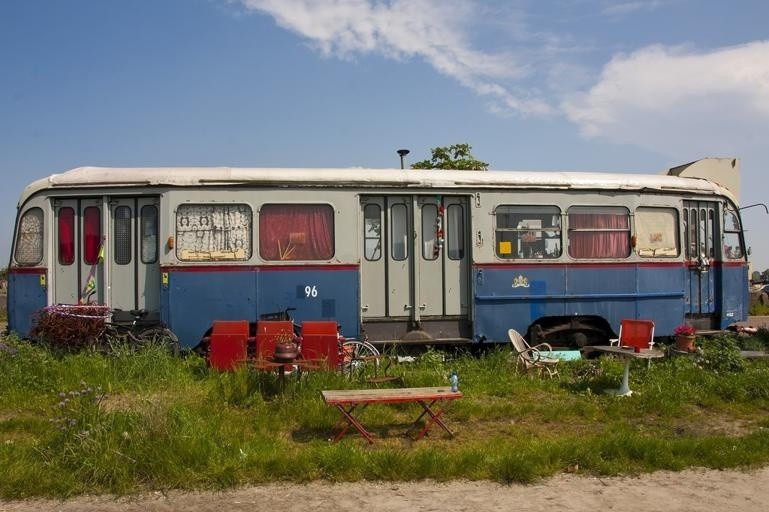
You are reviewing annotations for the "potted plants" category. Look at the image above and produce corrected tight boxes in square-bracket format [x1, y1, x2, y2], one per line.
[676, 336, 696, 352]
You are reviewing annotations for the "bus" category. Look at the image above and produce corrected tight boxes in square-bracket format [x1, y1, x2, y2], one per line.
[5, 148, 755, 355]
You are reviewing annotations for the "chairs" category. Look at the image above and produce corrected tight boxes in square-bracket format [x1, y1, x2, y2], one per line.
[507, 328, 560, 382]
[608, 319, 656, 371]
[203, 320, 345, 373]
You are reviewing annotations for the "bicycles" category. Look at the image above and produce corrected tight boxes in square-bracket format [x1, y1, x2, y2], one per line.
[280, 307, 382, 377]
[85, 290, 181, 361]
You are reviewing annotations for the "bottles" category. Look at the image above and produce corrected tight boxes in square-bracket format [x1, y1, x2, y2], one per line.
[451, 372, 459, 393]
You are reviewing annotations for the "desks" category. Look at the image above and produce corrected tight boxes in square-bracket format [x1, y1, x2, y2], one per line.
[583, 345, 665, 398]
[319, 386, 465, 445]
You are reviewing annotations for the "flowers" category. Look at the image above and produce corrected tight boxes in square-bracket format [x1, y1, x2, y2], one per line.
[674, 323, 697, 336]
